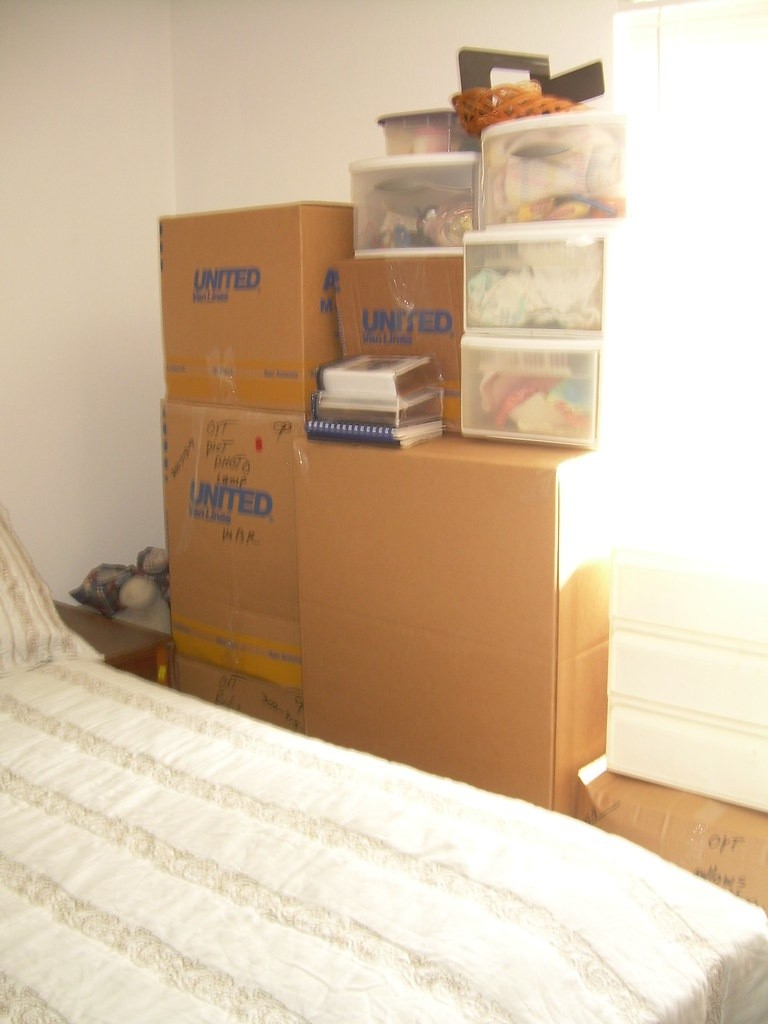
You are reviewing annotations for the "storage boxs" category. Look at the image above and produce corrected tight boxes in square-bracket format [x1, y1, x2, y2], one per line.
[159, 46, 631, 822]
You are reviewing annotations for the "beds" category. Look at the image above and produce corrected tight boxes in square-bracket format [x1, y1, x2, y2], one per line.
[0, 490, 768, 1024]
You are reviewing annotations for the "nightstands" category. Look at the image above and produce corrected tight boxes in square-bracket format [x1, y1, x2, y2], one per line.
[54, 599, 176, 689]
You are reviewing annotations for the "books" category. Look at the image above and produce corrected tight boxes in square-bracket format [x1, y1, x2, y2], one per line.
[306, 421, 443, 447]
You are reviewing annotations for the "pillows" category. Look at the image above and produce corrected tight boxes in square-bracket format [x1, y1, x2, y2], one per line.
[1, 498, 105, 682]
